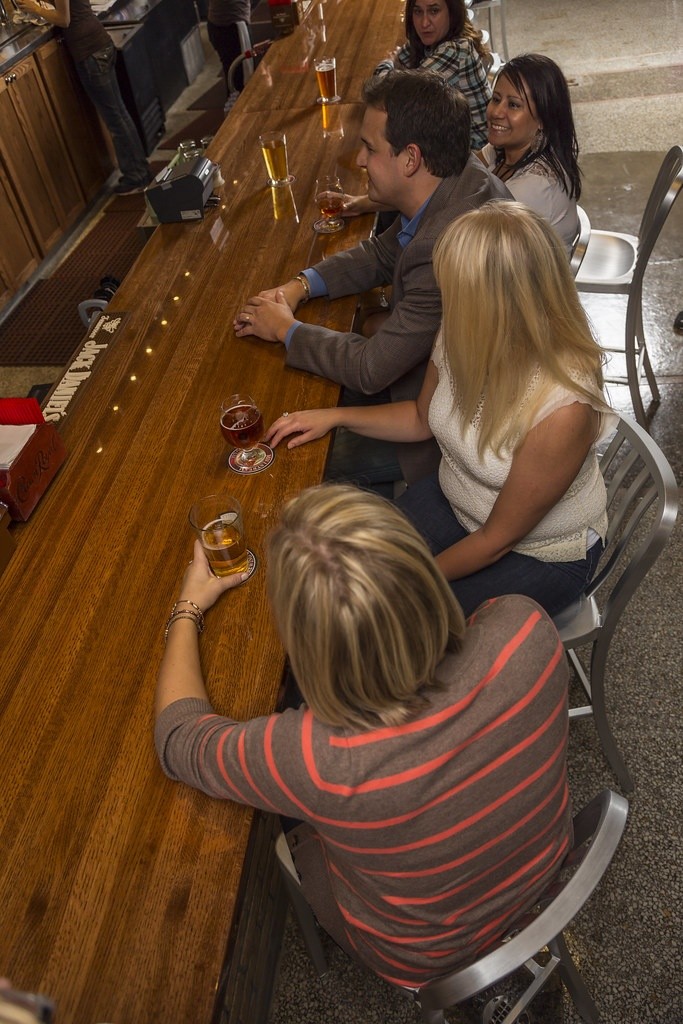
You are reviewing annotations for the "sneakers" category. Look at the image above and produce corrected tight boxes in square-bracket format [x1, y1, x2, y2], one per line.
[115, 172, 152, 196]
[117, 176, 130, 184]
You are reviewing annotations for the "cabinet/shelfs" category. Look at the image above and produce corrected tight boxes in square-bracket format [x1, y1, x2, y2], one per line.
[1, 31, 118, 319]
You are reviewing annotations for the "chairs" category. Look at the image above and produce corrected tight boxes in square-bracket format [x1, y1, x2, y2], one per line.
[271, 791, 630, 1023]
[573, 146, 683, 423]
[570, 200, 592, 286]
[546, 413, 679, 794]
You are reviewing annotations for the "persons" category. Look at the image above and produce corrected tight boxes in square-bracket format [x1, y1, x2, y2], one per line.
[153, 481, 576, 994]
[233, 69, 517, 487]
[17, 0, 155, 196]
[260, 196, 609, 617]
[206, 0, 254, 95]
[315, 54, 586, 340]
[360, 0, 498, 154]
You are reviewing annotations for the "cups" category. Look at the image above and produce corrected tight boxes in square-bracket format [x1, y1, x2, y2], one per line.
[258, 131, 291, 183]
[314, 57, 339, 104]
[270, 182, 298, 219]
[188, 495, 249, 578]
[322, 102, 346, 139]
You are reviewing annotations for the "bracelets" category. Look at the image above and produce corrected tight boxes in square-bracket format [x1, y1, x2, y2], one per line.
[161, 600, 206, 643]
[292, 276, 309, 304]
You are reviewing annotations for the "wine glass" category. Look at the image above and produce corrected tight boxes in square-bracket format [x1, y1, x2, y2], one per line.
[218, 390, 266, 469]
[312, 176, 345, 229]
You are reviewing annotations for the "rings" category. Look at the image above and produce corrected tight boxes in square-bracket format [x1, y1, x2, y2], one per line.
[246, 314, 250, 322]
[282, 412, 289, 418]
